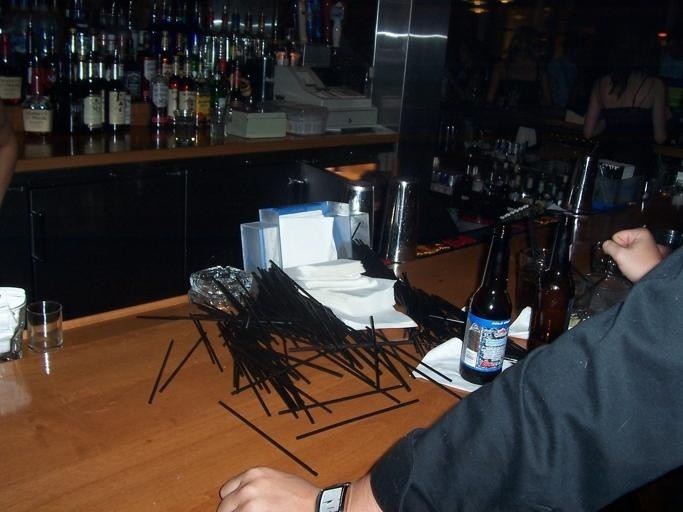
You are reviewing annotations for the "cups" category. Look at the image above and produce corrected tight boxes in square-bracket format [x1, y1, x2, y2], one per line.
[173, 109, 196, 144]
[210, 106, 233, 140]
[25, 300, 64, 352]
[0, 286, 28, 363]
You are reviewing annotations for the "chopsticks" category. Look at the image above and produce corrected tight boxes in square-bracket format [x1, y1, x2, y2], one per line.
[133, 257, 416, 482]
[350, 233, 464, 368]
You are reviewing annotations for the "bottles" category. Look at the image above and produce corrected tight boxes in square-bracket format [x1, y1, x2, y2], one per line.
[108, 48, 125, 136]
[244, 11, 254, 73]
[259, 7, 265, 31]
[430, 122, 574, 204]
[47, 27, 58, 88]
[169, 54, 179, 127]
[214, 63, 229, 134]
[0, 35, 22, 104]
[222, 3, 229, 33]
[99, 58, 106, 126]
[527, 215, 575, 355]
[151, 0, 202, 27]
[142, 35, 158, 101]
[83, 60, 102, 129]
[232, 3, 240, 31]
[286, 3, 295, 42]
[230, 54, 242, 92]
[1, 0, 133, 31]
[176, 33, 185, 75]
[151, 52, 169, 131]
[460, 221, 511, 384]
[22, 53, 53, 139]
[126, 38, 142, 101]
[196, 51, 213, 129]
[254, 13, 270, 65]
[184, 48, 192, 79]
[272, 6, 279, 40]
[203, 9, 219, 65]
[160, 31, 173, 78]
[231, 9, 241, 68]
[219, 3, 232, 64]
[175, 49, 195, 146]
[54, 58, 72, 147]
[253, 39, 275, 101]
[27, 23, 34, 90]
[246, 7, 252, 31]
[207, 3, 215, 33]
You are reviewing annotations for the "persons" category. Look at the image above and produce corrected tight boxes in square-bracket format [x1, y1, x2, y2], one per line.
[215, 226, 682, 511]
[0, 96, 20, 207]
[485, 25, 555, 110]
[583, 32, 669, 180]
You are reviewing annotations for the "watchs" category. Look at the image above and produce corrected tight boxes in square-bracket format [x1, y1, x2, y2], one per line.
[314, 482, 354, 512]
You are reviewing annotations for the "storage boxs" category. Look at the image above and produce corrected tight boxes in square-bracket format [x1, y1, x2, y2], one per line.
[593, 177, 635, 210]
[241, 220, 280, 273]
[337, 213, 371, 258]
